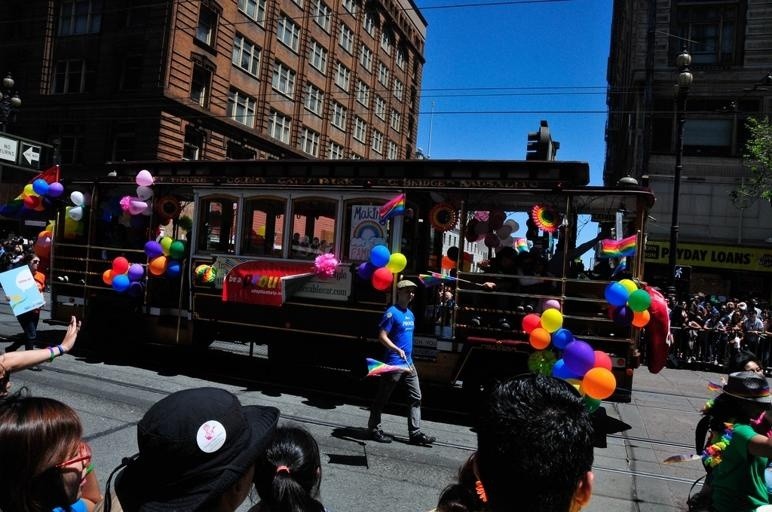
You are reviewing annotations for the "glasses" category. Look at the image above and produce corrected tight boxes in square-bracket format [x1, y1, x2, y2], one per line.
[37, 437, 93, 477]
[31, 260, 40, 264]
[403, 289, 415, 294]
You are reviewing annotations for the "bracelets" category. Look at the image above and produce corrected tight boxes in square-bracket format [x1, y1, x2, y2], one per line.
[56, 344, 64, 356]
[87, 464, 94, 474]
[46, 346, 54, 363]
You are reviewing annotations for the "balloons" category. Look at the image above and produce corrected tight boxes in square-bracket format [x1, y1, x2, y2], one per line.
[144, 237, 185, 278]
[522, 300, 617, 399]
[36, 220, 57, 258]
[359, 245, 407, 290]
[465, 209, 519, 248]
[22, 169, 153, 226]
[103, 256, 144, 292]
[605, 278, 650, 328]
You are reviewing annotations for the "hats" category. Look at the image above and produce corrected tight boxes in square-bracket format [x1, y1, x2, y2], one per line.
[723, 372, 772, 404]
[397, 280, 417, 288]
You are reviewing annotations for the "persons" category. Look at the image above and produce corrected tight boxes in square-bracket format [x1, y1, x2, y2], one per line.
[1, 385, 101, 511]
[291, 232, 335, 254]
[436, 451, 481, 512]
[641, 288, 770, 369]
[367, 280, 437, 444]
[474, 373, 595, 512]
[687, 371, 772, 512]
[436, 217, 627, 280]
[248, 423, 327, 510]
[4, 253, 46, 372]
[1, 314, 82, 392]
[1, 235, 36, 270]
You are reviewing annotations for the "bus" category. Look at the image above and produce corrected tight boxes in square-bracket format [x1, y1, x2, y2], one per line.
[48, 149, 657, 421]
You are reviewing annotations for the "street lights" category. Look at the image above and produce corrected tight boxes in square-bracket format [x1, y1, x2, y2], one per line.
[667, 45, 698, 285]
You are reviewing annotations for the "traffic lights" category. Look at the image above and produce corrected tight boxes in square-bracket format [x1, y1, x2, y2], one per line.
[672, 265, 693, 285]
[525, 119, 551, 160]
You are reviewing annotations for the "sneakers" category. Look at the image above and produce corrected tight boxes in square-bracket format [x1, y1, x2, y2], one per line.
[27, 366, 41, 372]
[366, 430, 392, 443]
[410, 433, 436, 446]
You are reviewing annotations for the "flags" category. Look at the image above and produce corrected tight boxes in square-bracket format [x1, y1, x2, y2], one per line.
[380, 192, 406, 226]
[364, 356, 411, 377]
[598, 235, 637, 256]
